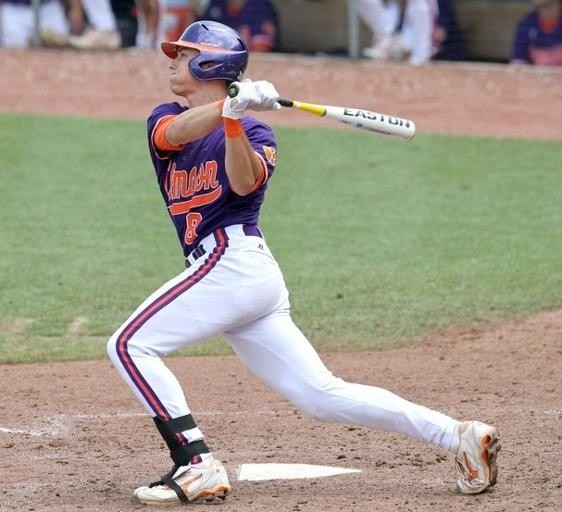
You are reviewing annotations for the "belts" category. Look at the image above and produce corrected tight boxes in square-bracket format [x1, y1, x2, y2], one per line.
[185, 226, 262, 269]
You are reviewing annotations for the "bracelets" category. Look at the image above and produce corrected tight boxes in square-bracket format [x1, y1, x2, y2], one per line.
[222, 117, 243, 140]
[217, 99, 225, 112]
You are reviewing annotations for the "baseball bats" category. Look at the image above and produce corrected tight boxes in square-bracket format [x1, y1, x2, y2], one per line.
[228, 84, 416, 140]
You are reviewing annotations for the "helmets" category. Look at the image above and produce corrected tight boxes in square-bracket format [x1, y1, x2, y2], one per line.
[161, 19, 249, 82]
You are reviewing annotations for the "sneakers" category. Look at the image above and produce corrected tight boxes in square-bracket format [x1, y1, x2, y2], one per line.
[454, 421, 502, 495]
[67, 26, 123, 51]
[131, 460, 231, 508]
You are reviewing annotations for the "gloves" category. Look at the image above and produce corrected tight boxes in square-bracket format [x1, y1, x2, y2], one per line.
[221, 81, 261, 120]
[248, 79, 282, 112]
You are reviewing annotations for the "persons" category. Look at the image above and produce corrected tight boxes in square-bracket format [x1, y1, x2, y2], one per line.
[353, 1, 460, 64]
[1, 1, 278, 52]
[509, 2, 562, 66]
[106, 20, 503, 507]
